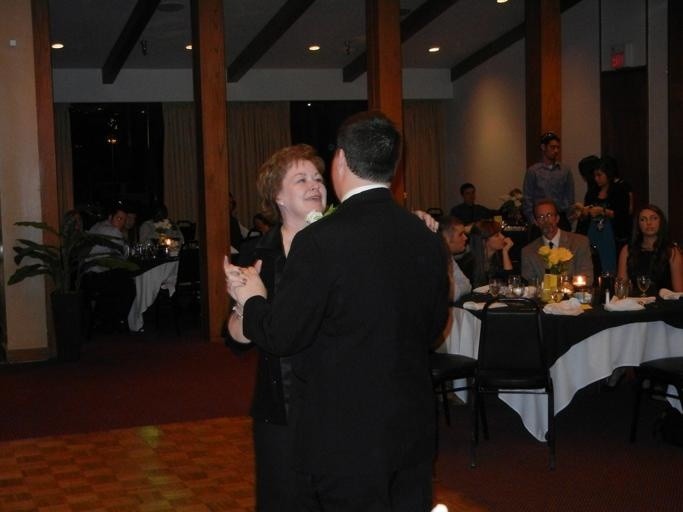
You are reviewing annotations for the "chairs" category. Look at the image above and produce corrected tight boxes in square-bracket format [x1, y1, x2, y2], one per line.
[469, 297, 555, 472]
[176, 218, 197, 241]
[426, 207, 443, 219]
[629, 299, 683, 443]
[154, 240, 199, 337]
[247, 227, 263, 238]
[427, 352, 490, 463]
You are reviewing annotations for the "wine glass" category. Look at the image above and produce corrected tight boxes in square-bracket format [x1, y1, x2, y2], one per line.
[489, 271, 615, 304]
[129, 238, 175, 258]
[635, 276, 650, 300]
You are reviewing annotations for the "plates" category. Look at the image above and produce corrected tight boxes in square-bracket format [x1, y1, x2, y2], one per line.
[544, 303, 580, 313]
[607, 302, 643, 312]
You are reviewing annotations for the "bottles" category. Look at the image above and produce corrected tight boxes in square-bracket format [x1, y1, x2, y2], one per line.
[615, 277, 629, 301]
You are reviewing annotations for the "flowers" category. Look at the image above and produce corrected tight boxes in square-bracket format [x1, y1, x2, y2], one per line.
[539, 247, 574, 274]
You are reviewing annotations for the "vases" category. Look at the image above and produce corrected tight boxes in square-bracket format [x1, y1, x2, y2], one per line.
[544, 274, 557, 290]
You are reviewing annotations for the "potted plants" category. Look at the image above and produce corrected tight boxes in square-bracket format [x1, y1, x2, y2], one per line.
[7, 219, 139, 346]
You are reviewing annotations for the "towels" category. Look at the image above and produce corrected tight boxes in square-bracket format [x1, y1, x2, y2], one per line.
[544, 301, 584, 317]
[604, 298, 645, 312]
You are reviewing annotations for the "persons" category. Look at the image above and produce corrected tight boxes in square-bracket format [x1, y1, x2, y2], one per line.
[80, 182, 683, 402]
[571, 158, 632, 274]
[523, 132, 575, 240]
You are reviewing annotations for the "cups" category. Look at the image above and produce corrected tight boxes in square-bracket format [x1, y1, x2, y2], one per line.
[494, 216, 523, 230]
